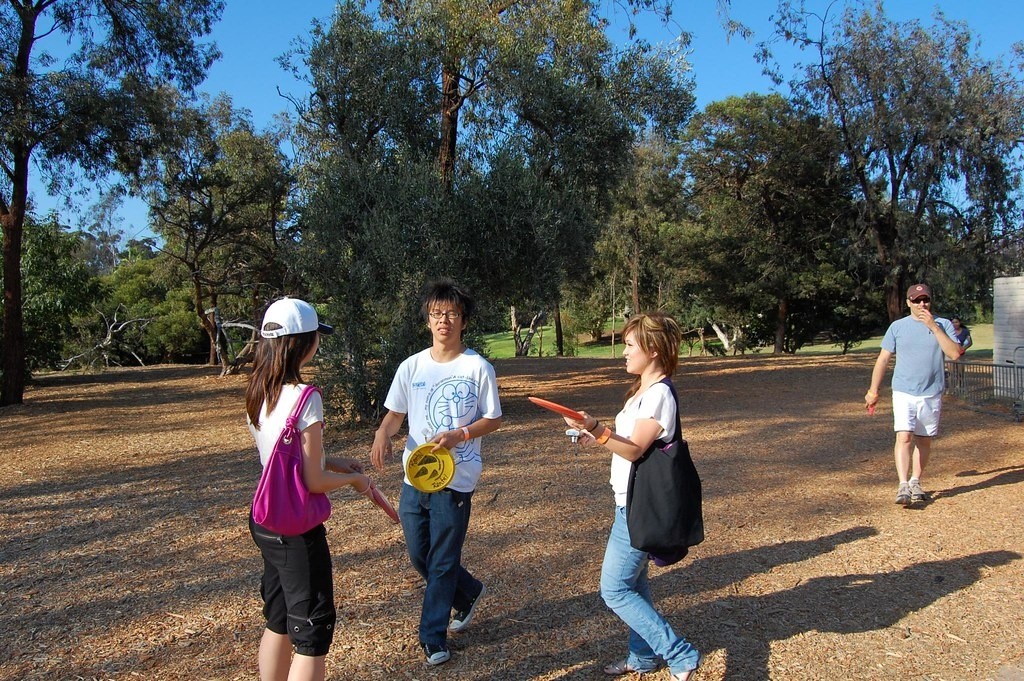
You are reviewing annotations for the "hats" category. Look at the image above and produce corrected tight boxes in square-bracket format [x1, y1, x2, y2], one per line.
[907, 284, 932, 301]
[260, 296, 334, 340]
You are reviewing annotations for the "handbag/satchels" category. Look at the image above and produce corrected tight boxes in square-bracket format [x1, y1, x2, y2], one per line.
[252, 386, 331, 536]
[625, 382, 706, 554]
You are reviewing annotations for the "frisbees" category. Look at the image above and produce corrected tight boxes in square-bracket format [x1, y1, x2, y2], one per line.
[371, 486, 402, 525]
[869, 404, 875, 416]
[528, 396, 584, 420]
[959, 348, 965, 354]
[405, 442, 456, 493]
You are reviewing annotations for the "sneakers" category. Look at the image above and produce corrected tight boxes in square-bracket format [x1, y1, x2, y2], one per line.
[419, 640, 451, 665]
[895, 483, 911, 504]
[909, 479, 927, 500]
[450, 582, 487, 632]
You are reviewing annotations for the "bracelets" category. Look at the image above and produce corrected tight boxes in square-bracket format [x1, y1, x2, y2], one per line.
[587, 419, 599, 432]
[596, 426, 612, 445]
[459, 426, 470, 441]
[867, 389, 878, 398]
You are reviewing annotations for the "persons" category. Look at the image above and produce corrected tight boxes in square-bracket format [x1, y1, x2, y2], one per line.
[246, 295, 380, 681]
[369, 280, 502, 666]
[864, 284, 961, 505]
[946, 317, 972, 394]
[562, 313, 705, 681]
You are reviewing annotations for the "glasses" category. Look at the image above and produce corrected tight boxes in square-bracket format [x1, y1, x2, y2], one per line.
[908, 297, 930, 304]
[428, 309, 464, 319]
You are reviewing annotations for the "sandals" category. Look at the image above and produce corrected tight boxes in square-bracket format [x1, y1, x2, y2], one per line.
[604, 658, 634, 675]
[671, 654, 703, 681]
